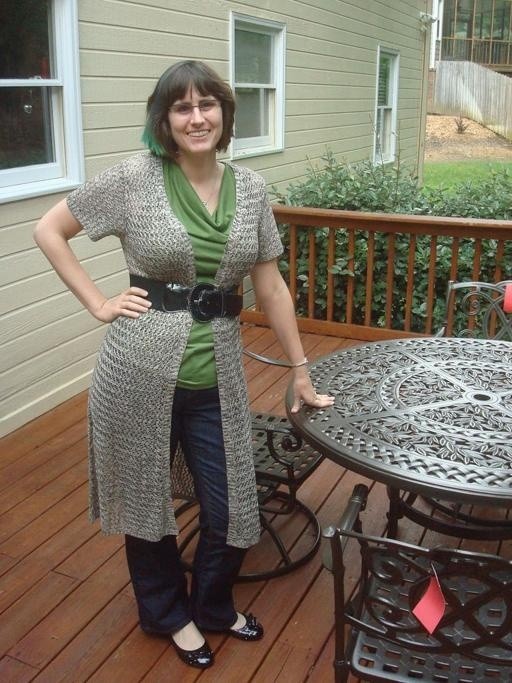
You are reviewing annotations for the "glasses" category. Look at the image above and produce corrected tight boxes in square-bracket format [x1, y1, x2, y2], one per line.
[172, 101, 213, 115]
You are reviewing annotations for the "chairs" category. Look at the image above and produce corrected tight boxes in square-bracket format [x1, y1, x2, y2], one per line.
[422, 285, 511, 523]
[323, 485, 510, 674]
[168, 349, 327, 583]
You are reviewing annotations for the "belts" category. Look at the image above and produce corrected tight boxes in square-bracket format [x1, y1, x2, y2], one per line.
[130, 274, 243, 323]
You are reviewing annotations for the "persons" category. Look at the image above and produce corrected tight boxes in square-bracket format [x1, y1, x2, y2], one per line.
[31, 62, 335, 667]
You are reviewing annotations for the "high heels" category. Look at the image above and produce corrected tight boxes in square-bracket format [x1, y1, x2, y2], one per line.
[168, 609, 263, 668]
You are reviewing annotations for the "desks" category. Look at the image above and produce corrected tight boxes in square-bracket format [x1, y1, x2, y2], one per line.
[285, 339, 510, 541]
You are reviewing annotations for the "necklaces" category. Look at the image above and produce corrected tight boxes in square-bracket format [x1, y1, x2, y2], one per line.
[174, 156, 219, 208]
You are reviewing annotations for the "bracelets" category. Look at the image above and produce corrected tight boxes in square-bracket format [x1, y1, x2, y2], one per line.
[293, 358, 308, 368]
